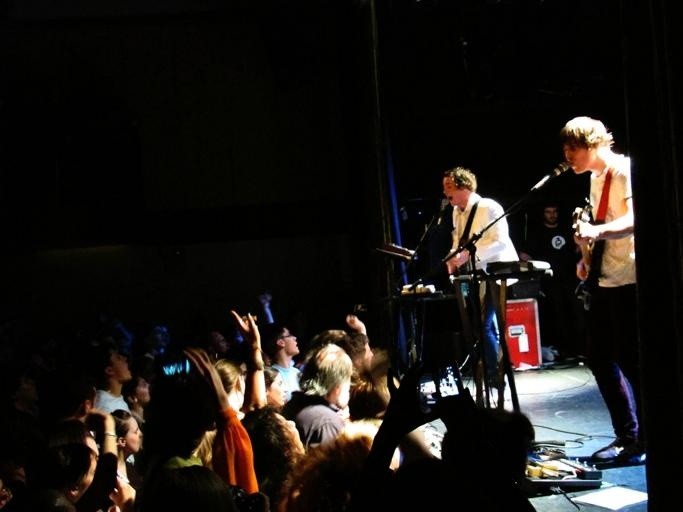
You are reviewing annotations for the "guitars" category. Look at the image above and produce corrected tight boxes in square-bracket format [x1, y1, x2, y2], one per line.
[572, 196, 593, 278]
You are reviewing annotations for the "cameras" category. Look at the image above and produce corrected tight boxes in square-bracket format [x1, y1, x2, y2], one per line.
[412, 362, 463, 404]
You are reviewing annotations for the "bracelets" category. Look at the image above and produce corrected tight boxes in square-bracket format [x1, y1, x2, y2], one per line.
[103, 431, 118, 438]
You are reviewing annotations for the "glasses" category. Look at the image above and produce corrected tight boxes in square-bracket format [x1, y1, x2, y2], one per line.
[279, 333, 292, 339]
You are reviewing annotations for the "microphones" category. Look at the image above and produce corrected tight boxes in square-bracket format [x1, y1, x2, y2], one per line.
[437, 198, 450, 227]
[532, 161, 569, 192]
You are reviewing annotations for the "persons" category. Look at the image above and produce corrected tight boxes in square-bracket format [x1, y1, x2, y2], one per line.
[337, 315, 375, 374]
[349, 378, 389, 420]
[231, 310, 306, 512]
[41, 370, 96, 427]
[3, 374, 46, 459]
[105, 318, 132, 350]
[134, 465, 271, 512]
[442, 167, 519, 405]
[519, 201, 581, 369]
[261, 323, 302, 401]
[111, 410, 144, 512]
[144, 348, 258, 495]
[562, 117, 647, 467]
[209, 330, 229, 362]
[122, 374, 151, 431]
[53, 376, 118, 511]
[193, 358, 245, 469]
[282, 344, 353, 452]
[1, 420, 97, 512]
[93, 343, 132, 411]
[282, 437, 399, 512]
[260, 292, 275, 324]
[263, 367, 288, 412]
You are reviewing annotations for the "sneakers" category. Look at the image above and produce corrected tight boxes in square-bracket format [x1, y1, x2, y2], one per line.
[616, 448, 647, 464]
[484, 369, 506, 390]
[589, 439, 623, 465]
